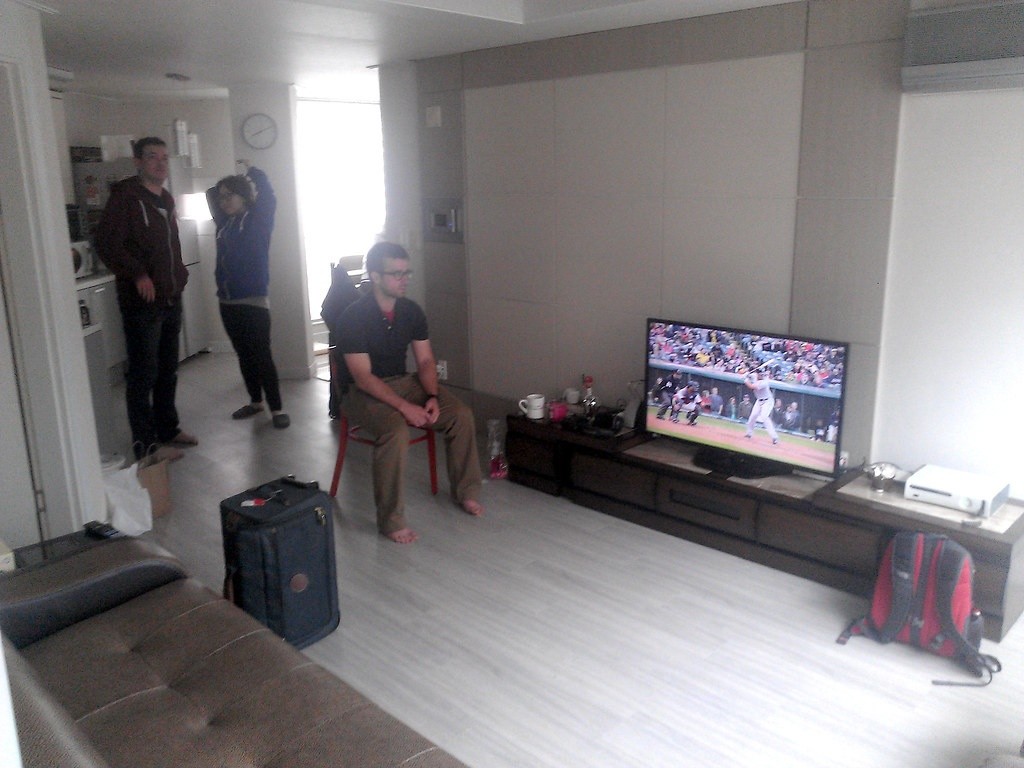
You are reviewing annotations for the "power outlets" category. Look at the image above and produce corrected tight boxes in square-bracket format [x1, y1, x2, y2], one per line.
[435, 360, 449, 380]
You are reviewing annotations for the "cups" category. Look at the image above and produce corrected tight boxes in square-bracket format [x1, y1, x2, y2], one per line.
[519, 393, 545, 420]
[563, 387, 580, 404]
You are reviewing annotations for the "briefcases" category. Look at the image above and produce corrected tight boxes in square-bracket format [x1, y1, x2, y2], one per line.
[217, 475, 341, 654]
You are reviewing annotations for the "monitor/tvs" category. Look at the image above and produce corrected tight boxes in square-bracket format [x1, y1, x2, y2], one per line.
[644, 317, 851, 479]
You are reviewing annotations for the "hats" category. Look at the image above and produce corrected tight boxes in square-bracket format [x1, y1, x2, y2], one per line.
[757, 368, 769, 375]
[672, 369, 684, 376]
[689, 381, 700, 391]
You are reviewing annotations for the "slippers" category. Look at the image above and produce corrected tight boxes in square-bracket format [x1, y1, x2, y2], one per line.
[174, 432, 199, 447]
[233, 404, 263, 419]
[273, 411, 290, 428]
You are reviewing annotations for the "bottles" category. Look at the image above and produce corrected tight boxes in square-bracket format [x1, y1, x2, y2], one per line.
[79, 299, 90, 327]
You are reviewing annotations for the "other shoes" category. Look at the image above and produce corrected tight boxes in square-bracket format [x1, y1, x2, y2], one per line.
[745, 434, 753, 440]
[657, 414, 665, 420]
[670, 417, 678, 423]
[772, 439, 779, 443]
[687, 421, 696, 426]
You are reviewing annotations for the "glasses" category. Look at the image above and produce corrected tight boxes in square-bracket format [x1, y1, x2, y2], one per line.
[378, 268, 413, 280]
[216, 192, 236, 202]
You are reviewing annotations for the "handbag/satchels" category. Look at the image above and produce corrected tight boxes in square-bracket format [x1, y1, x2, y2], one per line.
[97, 464, 153, 536]
[128, 439, 171, 520]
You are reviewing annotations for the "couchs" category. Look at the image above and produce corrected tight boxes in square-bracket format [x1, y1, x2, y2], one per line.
[0, 525, 475, 768]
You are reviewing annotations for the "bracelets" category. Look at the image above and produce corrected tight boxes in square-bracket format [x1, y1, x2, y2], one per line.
[426, 394, 439, 400]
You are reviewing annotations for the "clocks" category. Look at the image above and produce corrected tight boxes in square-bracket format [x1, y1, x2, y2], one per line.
[241, 112, 278, 151]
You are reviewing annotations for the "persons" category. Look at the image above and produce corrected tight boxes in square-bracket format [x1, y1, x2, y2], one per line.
[93, 137, 198, 462]
[204, 158, 290, 430]
[650, 321, 846, 450]
[334, 239, 483, 545]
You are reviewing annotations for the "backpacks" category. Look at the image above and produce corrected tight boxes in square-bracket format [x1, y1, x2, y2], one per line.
[834, 531, 1000, 688]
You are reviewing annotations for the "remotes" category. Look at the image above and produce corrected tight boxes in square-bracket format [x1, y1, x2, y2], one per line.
[82, 520, 136, 540]
